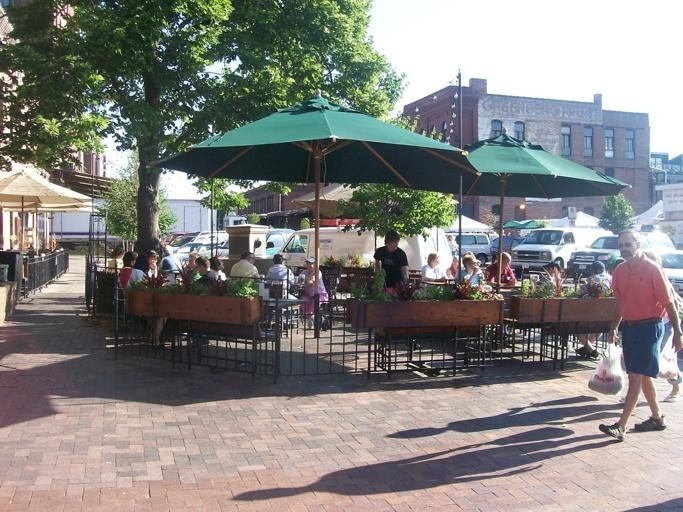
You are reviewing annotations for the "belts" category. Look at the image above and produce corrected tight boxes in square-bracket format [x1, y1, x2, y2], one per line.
[626, 317, 664, 327]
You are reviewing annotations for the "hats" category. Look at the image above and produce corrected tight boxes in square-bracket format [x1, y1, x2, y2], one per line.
[383, 230, 401, 243]
[303, 256, 315, 264]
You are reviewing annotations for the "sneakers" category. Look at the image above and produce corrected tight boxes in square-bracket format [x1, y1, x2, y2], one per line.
[633, 413, 667, 435]
[573, 347, 586, 357]
[616, 394, 628, 405]
[596, 422, 627, 443]
[662, 390, 680, 402]
[590, 349, 600, 358]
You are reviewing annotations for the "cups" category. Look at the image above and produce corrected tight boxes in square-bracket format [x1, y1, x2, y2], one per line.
[373, 259, 381, 273]
[288, 274, 305, 285]
[500, 273, 517, 286]
[259, 275, 264, 282]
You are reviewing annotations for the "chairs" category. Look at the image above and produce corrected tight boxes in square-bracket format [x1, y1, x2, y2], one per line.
[89, 264, 523, 356]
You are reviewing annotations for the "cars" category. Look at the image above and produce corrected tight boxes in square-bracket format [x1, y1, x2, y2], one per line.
[647, 247, 682, 297]
[158, 224, 300, 278]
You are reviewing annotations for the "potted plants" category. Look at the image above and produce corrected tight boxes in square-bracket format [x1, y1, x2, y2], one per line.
[509, 262, 617, 325]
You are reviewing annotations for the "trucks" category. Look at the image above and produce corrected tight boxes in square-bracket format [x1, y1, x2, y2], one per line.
[277, 223, 454, 309]
[47, 198, 248, 259]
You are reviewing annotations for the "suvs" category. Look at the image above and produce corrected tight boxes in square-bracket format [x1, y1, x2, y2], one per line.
[444, 228, 675, 285]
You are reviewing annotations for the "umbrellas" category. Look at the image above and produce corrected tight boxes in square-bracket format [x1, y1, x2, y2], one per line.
[0, 170, 101, 251]
[146, 88, 482, 337]
[462, 128, 633, 293]
[518, 220, 544, 228]
[446, 215, 489, 232]
[492, 220, 521, 235]
[291, 182, 361, 219]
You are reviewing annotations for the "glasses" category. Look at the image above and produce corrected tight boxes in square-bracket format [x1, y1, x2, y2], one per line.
[617, 241, 638, 249]
